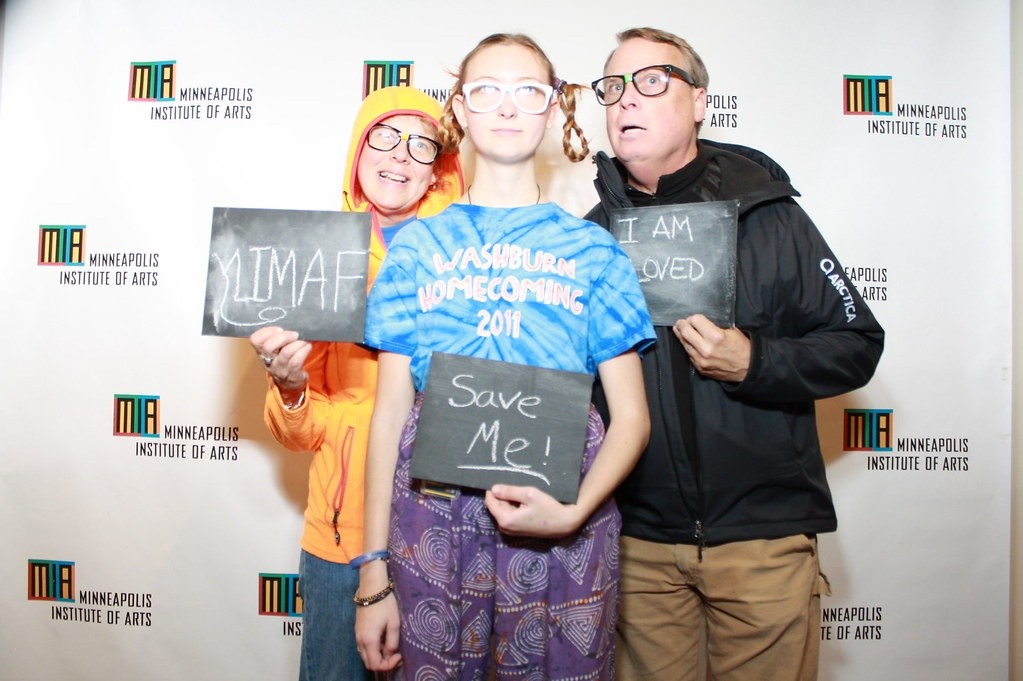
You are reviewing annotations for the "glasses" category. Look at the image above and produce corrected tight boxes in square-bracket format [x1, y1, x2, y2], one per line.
[460, 81, 554, 114]
[366, 123, 443, 165]
[591, 65, 697, 106]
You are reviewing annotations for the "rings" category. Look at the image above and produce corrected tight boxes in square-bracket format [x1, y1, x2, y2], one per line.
[263, 358, 274, 366]
[357, 644, 365, 652]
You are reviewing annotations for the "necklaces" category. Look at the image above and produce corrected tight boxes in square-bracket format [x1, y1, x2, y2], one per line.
[468, 184, 540, 205]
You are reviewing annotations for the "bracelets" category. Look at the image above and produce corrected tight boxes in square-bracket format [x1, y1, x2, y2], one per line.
[350, 550, 390, 570]
[353, 577, 395, 607]
[285, 390, 304, 409]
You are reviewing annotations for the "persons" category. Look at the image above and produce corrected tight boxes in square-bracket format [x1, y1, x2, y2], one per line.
[249, 88, 464, 681]
[580, 28, 885, 681]
[349, 33, 656, 681]
